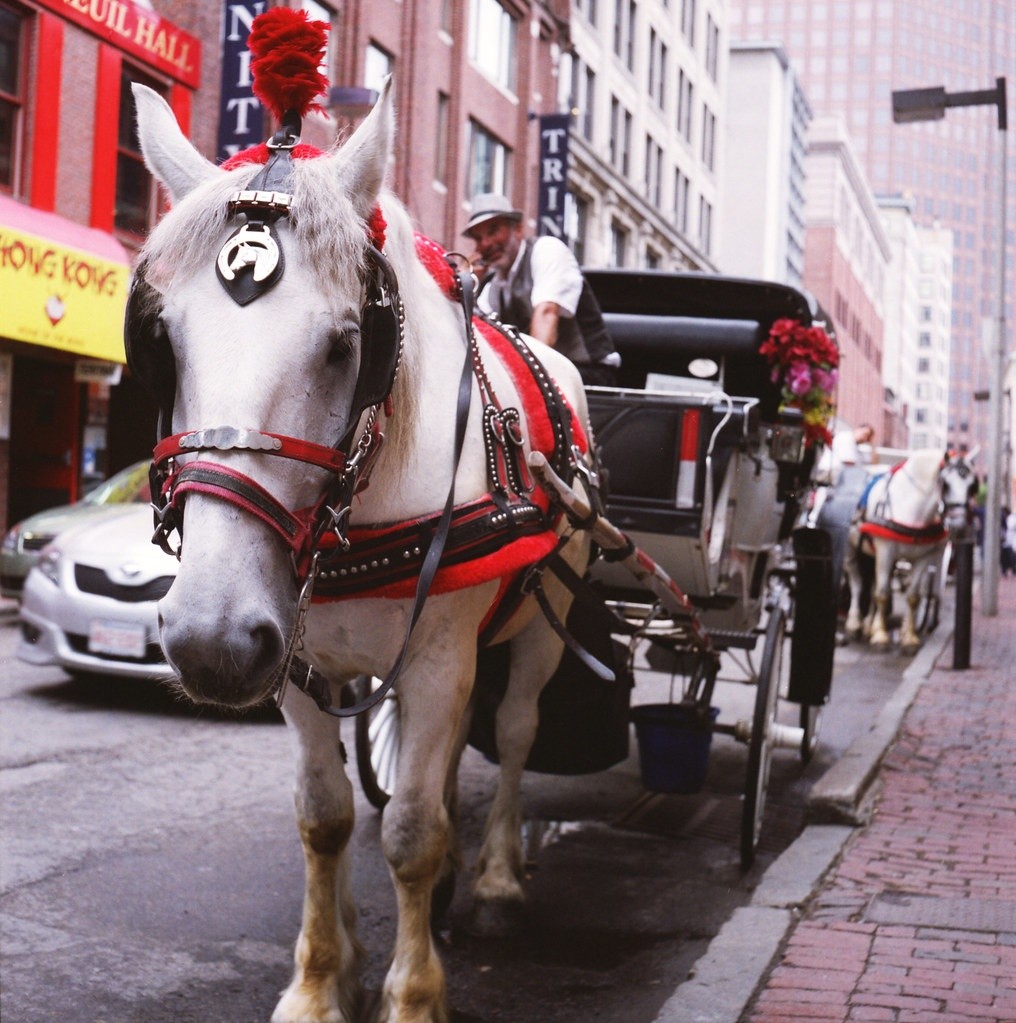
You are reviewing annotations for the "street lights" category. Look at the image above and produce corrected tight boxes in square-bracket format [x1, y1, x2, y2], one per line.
[892, 74, 1008, 617]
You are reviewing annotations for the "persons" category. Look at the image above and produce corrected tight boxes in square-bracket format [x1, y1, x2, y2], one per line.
[458, 192, 621, 386]
[814, 423, 876, 486]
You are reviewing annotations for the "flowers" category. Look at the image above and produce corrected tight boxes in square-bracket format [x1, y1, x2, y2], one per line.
[759, 318, 841, 450]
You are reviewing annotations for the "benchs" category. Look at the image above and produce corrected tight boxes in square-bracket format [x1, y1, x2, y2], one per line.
[579, 311, 763, 505]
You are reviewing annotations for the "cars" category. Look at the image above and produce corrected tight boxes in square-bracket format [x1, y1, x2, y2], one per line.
[1, 458, 154, 591]
[16, 502, 180, 690]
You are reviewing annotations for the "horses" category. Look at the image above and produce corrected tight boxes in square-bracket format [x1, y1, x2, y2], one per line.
[130, 73, 609, 1023]
[838, 442, 982, 656]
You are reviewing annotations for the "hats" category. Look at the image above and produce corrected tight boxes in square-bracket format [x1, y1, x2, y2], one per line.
[461, 193, 523, 238]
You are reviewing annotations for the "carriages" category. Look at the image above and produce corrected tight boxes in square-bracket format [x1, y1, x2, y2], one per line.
[129, 2, 843, 1023]
[797, 442, 983, 657]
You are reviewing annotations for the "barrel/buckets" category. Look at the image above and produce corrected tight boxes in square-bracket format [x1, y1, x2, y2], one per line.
[633, 703, 720, 795]
